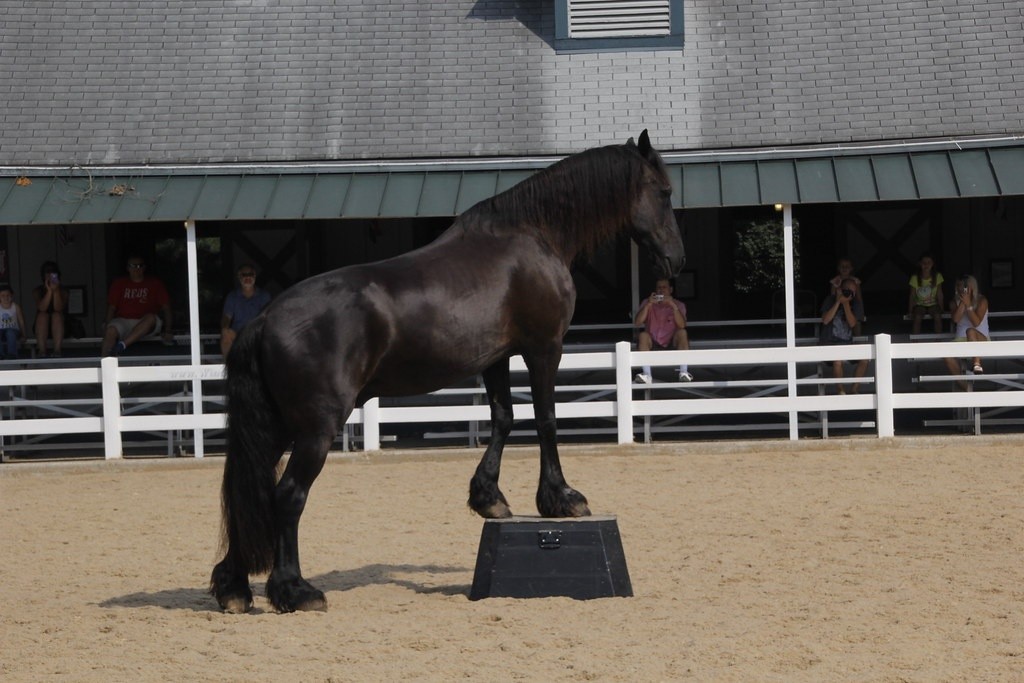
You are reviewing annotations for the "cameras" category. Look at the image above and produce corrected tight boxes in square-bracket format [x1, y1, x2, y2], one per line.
[656, 295, 664, 301]
[964, 288, 967, 294]
[50, 273, 58, 280]
[842, 290, 854, 298]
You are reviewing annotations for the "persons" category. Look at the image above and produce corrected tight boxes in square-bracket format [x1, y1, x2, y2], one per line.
[222, 262, 273, 359]
[0, 285, 27, 360]
[102, 255, 175, 355]
[821, 258, 869, 393]
[908, 254, 946, 341]
[633, 275, 692, 383]
[943, 274, 992, 391]
[32, 266, 77, 358]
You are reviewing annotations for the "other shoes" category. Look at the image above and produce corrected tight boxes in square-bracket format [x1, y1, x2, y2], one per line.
[967, 383, 973, 392]
[973, 364, 983, 374]
[635, 374, 652, 385]
[679, 372, 693, 382]
[116, 340, 125, 353]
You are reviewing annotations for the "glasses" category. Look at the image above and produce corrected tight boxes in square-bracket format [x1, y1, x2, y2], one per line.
[129, 263, 143, 269]
[237, 273, 253, 277]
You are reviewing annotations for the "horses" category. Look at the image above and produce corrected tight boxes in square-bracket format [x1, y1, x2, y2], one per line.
[205, 125, 689, 617]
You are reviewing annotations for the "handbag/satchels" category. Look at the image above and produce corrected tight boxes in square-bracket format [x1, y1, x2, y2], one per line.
[68, 319, 85, 339]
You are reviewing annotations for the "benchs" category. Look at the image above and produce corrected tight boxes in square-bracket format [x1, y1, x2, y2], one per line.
[0, 333, 226, 451]
[381, 311, 1024, 441]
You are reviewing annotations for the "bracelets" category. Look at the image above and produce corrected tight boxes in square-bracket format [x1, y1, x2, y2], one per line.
[966, 306, 973, 310]
